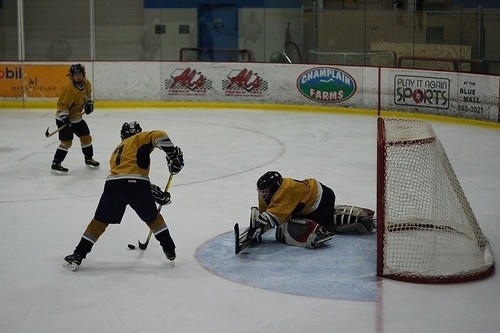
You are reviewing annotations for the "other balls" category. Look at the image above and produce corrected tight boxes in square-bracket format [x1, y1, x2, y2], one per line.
[128, 244, 135, 250]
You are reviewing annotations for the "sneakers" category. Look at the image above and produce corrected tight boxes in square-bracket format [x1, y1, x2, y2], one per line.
[164, 244, 176, 267]
[63, 254, 83, 272]
[49, 161, 69, 176]
[85, 157, 99, 168]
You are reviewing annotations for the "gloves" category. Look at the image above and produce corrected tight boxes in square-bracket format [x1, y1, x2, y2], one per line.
[62, 116, 72, 127]
[86, 101, 94, 114]
[166, 146, 184, 176]
[248, 224, 264, 238]
[150, 184, 172, 205]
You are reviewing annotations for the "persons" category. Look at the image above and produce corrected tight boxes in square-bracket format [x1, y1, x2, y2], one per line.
[63, 122, 185, 271]
[51, 64, 100, 175]
[248, 172, 376, 249]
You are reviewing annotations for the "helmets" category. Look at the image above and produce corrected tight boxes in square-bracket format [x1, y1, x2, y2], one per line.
[259, 173, 282, 203]
[121, 121, 141, 139]
[69, 64, 85, 82]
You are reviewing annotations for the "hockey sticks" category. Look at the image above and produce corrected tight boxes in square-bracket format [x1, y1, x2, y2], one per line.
[45, 111, 86, 137]
[233, 223, 259, 255]
[138, 174, 174, 250]
